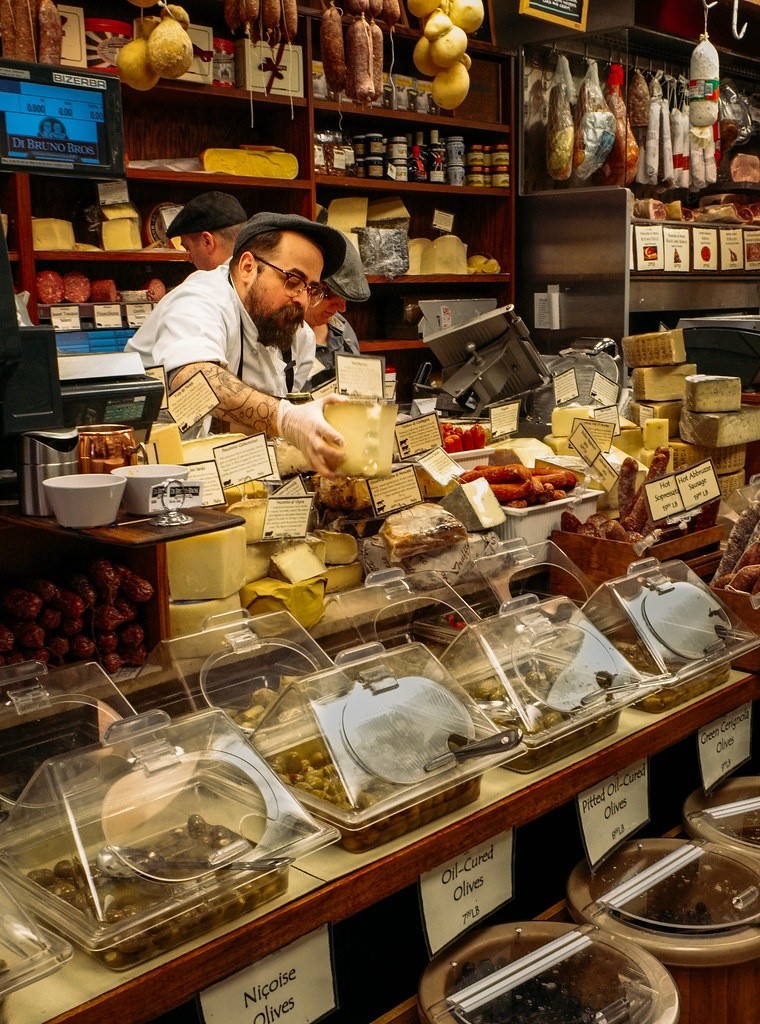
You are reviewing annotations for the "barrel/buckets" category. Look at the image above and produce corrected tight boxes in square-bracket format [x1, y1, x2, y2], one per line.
[84, 18, 133, 75]
[212, 37, 235, 89]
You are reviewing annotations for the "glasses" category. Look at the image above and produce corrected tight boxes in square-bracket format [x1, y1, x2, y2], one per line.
[254, 256, 328, 308]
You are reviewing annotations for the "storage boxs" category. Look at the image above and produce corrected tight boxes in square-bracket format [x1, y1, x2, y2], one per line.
[482, 488, 605, 567]
[237, 37, 306, 97]
[176, 24, 214, 83]
[553, 522, 731, 602]
[55, 5, 88, 67]
[395, 447, 496, 471]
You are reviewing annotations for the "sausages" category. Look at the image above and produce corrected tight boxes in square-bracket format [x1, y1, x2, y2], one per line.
[459, 464, 577, 508]
[709, 500, 760, 595]
[224, 0, 403, 108]
[560, 447, 721, 542]
[33, 271, 167, 304]
[0, 535, 156, 671]
[438, 422, 487, 452]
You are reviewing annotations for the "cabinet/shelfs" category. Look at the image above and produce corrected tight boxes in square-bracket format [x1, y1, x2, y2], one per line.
[0, 0, 520, 352]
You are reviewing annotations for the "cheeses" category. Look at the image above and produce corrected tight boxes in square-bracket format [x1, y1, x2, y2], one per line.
[436, 477, 506, 531]
[312, 195, 468, 277]
[499, 326, 760, 511]
[145, 397, 408, 658]
[29, 201, 183, 250]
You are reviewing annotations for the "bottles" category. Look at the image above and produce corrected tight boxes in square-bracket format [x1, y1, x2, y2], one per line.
[406, 130, 446, 184]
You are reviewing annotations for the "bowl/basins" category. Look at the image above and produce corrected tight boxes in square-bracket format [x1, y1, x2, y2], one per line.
[42, 474, 127, 528]
[111, 465, 190, 514]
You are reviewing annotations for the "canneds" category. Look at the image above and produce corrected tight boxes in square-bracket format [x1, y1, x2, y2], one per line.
[464, 144, 510, 187]
[351, 133, 407, 183]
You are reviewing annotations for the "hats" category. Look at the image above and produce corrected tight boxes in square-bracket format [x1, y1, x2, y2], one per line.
[165, 192, 247, 238]
[322, 229, 371, 301]
[233, 212, 347, 281]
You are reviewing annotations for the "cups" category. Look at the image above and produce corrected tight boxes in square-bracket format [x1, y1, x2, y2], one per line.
[383, 85, 393, 110]
[407, 89, 418, 112]
[76, 424, 150, 475]
[427, 94, 438, 115]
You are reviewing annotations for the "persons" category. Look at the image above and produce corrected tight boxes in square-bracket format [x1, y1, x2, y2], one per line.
[166, 193, 248, 271]
[301, 232, 371, 394]
[123, 211, 349, 481]
